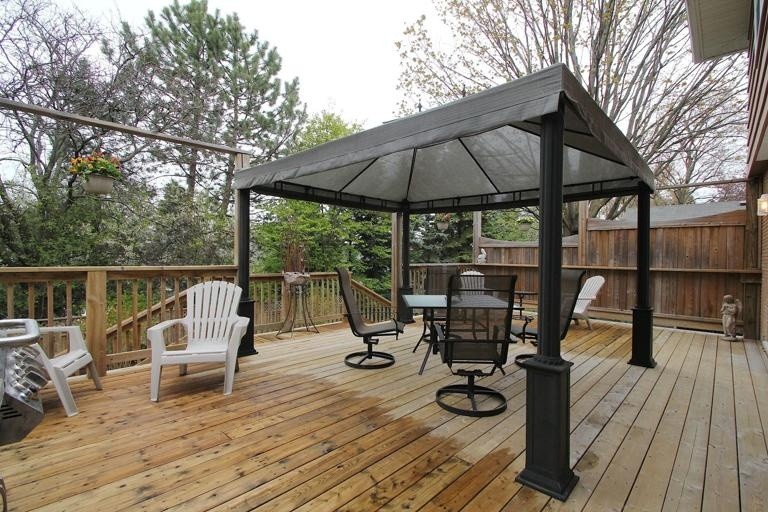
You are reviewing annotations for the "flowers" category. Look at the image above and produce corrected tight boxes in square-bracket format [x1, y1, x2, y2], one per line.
[71, 150, 123, 182]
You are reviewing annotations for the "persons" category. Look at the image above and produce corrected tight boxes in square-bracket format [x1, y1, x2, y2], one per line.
[719, 294, 739, 339]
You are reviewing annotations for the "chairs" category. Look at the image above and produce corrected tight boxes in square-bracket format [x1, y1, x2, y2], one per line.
[460, 269, 486, 295]
[433, 274, 519, 416]
[334, 266, 404, 370]
[144, 280, 250, 403]
[561, 274, 604, 331]
[509, 270, 588, 368]
[412, 266, 458, 353]
[0, 281, 104, 416]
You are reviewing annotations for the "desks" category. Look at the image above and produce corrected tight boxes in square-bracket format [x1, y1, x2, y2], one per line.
[401, 294, 526, 377]
[510, 287, 538, 322]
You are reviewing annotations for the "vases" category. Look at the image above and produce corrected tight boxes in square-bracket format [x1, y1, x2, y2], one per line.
[82, 172, 117, 195]
